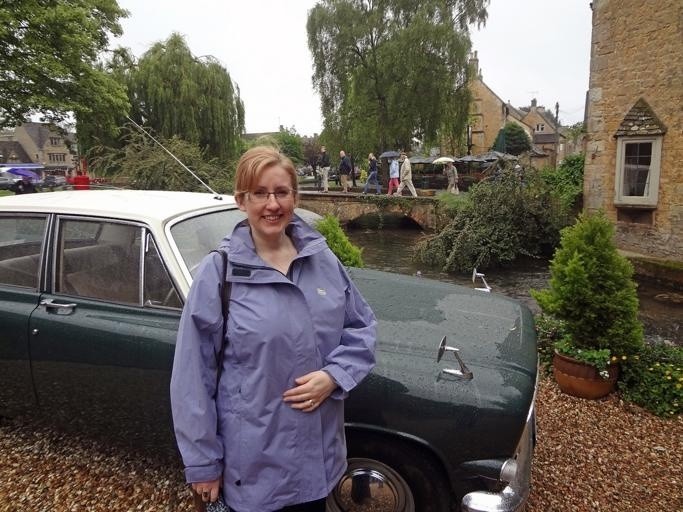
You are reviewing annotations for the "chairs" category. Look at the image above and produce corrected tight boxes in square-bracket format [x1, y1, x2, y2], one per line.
[62, 260, 153, 305]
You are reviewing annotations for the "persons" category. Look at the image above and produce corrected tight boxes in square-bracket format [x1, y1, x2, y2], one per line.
[442, 161, 459, 193]
[6, 159, 89, 194]
[318, 145, 417, 199]
[169, 146, 374, 512]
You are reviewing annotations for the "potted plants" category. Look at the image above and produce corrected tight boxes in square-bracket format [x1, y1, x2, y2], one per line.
[529, 206, 646, 400]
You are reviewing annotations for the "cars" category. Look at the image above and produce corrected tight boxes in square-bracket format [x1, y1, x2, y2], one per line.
[0, 116, 540, 511]
[1, 167, 70, 192]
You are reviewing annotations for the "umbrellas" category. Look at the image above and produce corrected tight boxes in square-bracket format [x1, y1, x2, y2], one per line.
[432, 157, 456, 173]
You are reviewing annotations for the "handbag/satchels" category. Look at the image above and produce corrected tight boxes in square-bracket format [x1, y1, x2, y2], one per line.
[200, 487, 231, 512]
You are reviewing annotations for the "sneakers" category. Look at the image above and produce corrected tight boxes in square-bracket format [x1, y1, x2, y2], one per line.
[321, 189, 420, 198]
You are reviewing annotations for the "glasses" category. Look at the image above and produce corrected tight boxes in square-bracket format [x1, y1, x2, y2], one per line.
[239, 189, 297, 201]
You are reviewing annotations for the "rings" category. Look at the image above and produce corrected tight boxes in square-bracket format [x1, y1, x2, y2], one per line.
[309, 399, 315, 407]
[202, 492, 209, 497]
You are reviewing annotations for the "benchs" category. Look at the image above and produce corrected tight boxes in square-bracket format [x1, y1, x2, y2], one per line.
[0, 242, 127, 296]
